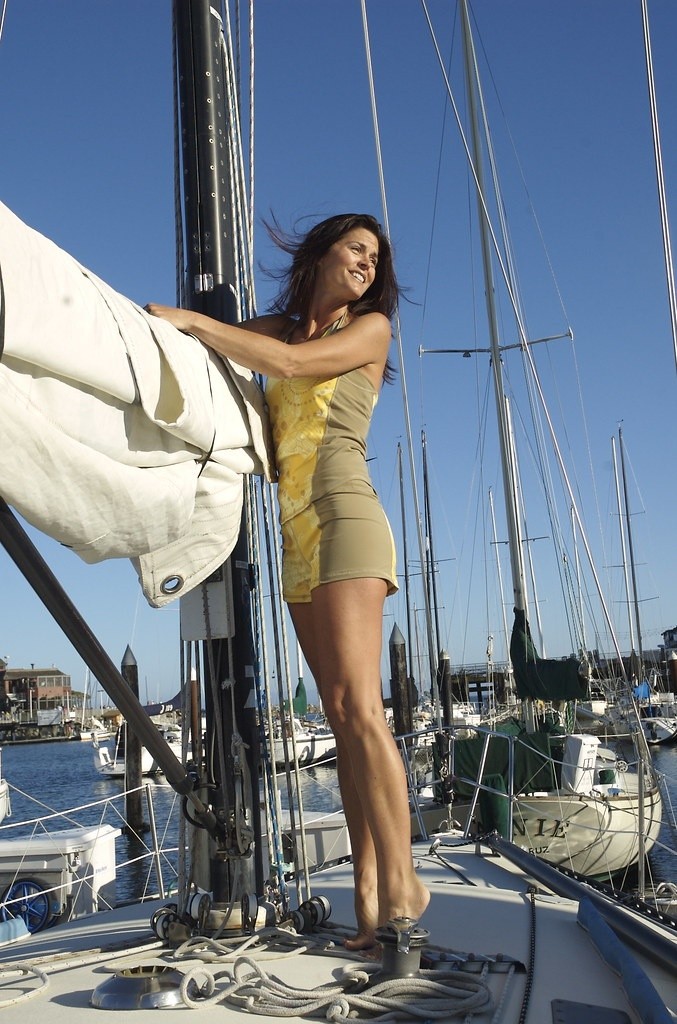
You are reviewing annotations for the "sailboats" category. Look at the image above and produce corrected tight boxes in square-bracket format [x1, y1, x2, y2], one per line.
[1, 2, 676, 939]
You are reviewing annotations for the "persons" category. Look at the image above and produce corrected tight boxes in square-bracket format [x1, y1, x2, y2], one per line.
[141, 211, 430, 948]
